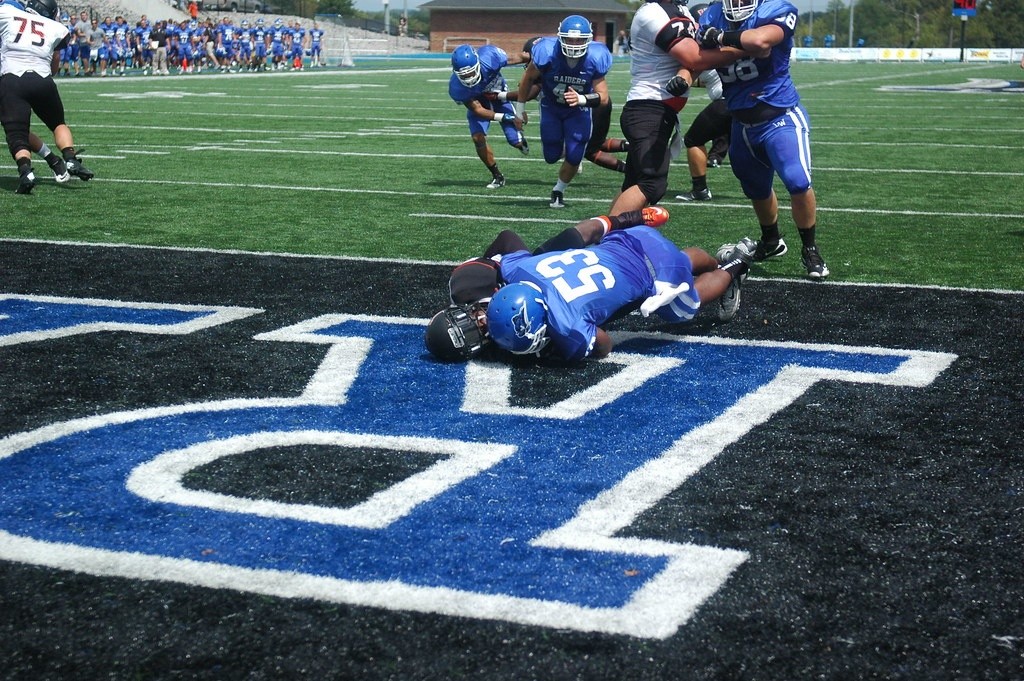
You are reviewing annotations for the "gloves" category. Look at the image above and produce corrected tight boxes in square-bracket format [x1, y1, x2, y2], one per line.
[665, 75, 690, 96]
[700, 25, 723, 48]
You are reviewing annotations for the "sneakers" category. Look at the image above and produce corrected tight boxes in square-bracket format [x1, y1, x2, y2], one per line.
[675, 188, 712, 201]
[716, 242, 738, 266]
[706, 159, 719, 168]
[48, 156, 71, 182]
[801, 246, 830, 277]
[65, 161, 94, 181]
[718, 270, 749, 323]
[618, 207, 669, 227]
[518, 131, 529, 155]
[17, 171, 35, 194]
[730, 236, 757, 282]
[752, 235, 788, 262]
[487, 175, 505, 188]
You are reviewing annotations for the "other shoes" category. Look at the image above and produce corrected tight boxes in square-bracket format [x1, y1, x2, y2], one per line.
[549, 190, 564, 207]
[62, 60, 323, 77]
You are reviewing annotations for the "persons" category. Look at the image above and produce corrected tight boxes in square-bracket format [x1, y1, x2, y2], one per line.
[189, 0, 198, 20]
[856, 36, 864, 47]
[425, 206, 756, 361]
[803, 33, 813, 46]
[610, 0, 772, 215]
[449, 45, 529, 189]
[667, 0, 829, 279]
[676, 5, 730, 202]
[56, 12, 324, 76]
[485, 15, 630, 210]
[825, 35, 832, 47]
[1, 0, 92, 193]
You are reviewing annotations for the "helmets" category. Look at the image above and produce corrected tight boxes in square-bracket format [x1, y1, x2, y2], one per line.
[28, 0, 57, 21]
[486, 282, 551, 354]
[425, 305, 490, 356]
[688, 3, 710, 23]
[451, 44, 481, 88]
[557, 15, 593, 58]
